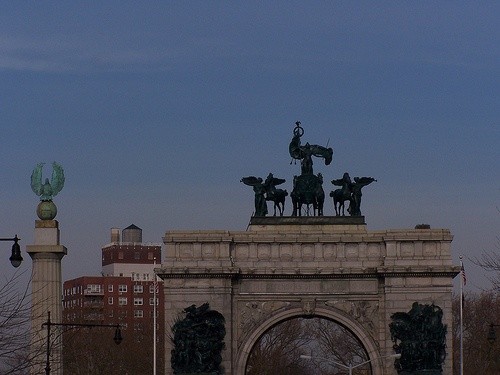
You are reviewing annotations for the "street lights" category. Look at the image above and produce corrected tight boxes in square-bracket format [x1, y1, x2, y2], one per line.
[297, 350, 403, 375]
[47, 315, 122, 375]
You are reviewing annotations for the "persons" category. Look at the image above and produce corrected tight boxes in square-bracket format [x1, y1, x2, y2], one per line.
[244, 132, 376, 216]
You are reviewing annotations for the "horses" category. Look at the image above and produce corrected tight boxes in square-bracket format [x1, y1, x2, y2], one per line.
[263, 172, 289, 217]
[329, 173, 361, 218]
